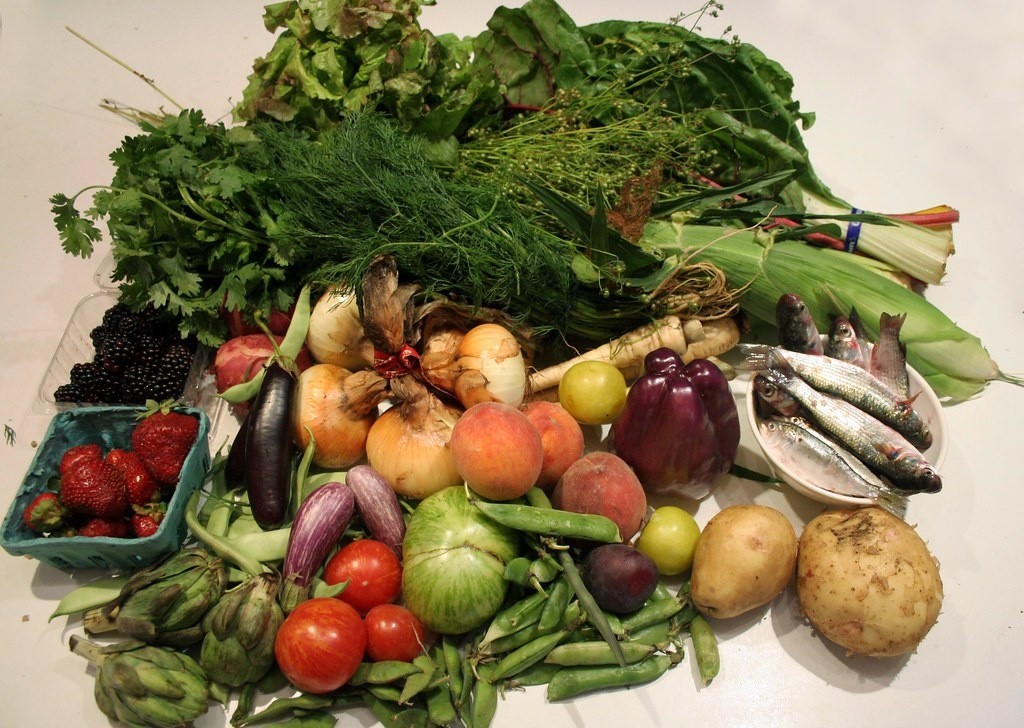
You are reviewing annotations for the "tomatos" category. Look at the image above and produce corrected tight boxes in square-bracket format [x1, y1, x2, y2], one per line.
[275, 597, 367, 694]
[322, 540, 405, 615]
[363, 603, 424, 663]
[400, 485, 518, 635]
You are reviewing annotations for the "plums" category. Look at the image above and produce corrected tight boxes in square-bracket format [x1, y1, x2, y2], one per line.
[582, 545, 660, 615]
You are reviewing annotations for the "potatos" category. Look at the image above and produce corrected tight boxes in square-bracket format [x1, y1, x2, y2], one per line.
[689, 505, 944, 656]
[211, 304, 318, 420]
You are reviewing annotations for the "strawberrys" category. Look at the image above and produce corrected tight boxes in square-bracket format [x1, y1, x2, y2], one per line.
[22, 400, 199, 541]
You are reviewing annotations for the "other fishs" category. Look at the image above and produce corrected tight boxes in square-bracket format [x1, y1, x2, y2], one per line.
[735, 293, 943, 522]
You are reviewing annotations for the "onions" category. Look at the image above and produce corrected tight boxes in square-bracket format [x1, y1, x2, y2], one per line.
[286, 270, 527, 499]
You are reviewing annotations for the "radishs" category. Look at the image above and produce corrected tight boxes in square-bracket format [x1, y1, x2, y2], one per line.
[523, 308, 741, 408]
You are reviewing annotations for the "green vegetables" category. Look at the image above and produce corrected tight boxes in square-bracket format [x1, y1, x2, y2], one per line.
[48, 0, 960, 340]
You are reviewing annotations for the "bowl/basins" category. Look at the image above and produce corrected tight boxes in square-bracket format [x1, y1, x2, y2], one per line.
[746, 334, 949, 510]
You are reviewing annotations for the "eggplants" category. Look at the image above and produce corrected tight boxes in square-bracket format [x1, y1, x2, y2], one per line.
[281, 481, 354, 615]
[347, 465, 405, 562]
[223, 411, 252, 492]
[245, 357, 303, 532]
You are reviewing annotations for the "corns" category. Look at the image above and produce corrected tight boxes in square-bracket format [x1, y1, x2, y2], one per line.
[616, 214, 1001, 399]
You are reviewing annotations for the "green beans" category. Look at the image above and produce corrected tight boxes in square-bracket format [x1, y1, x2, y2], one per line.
[213, 283, 311, 403]
[45, 426, 719, 728]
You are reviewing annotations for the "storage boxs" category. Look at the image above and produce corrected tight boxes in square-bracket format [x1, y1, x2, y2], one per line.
[38, 291, 208, 413]
[0, 405, 210, 570]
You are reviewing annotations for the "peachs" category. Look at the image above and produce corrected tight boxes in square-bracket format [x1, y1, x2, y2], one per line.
[551, 451, 645, 545]
[448, 402, 542, 499]
[518, 401, 584, 487]
